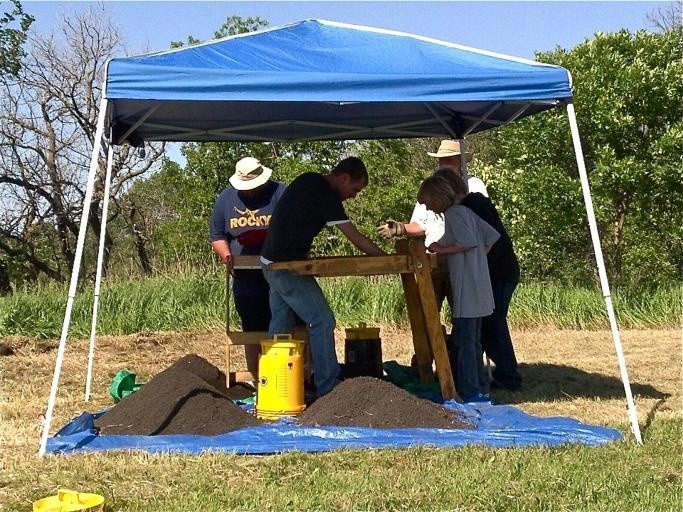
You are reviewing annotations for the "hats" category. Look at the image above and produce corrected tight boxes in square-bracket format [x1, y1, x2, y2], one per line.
[428, 140, 472, 164]
[229, 157, 272, 191]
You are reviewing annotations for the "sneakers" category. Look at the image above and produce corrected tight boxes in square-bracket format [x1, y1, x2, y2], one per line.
[489, 380, 520, 391]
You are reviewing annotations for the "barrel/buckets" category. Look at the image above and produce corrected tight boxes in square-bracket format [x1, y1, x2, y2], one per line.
[345, 338, 382, 378]
[257, 339, 305, 418]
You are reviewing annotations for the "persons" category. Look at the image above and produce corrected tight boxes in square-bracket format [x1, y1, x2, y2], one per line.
[416, 172, 502, 407]
[259, 156, 388, 398]
[432, 164, 524, 391]
[375, 139, 490, 248]
[208, 156, 288, 332]
